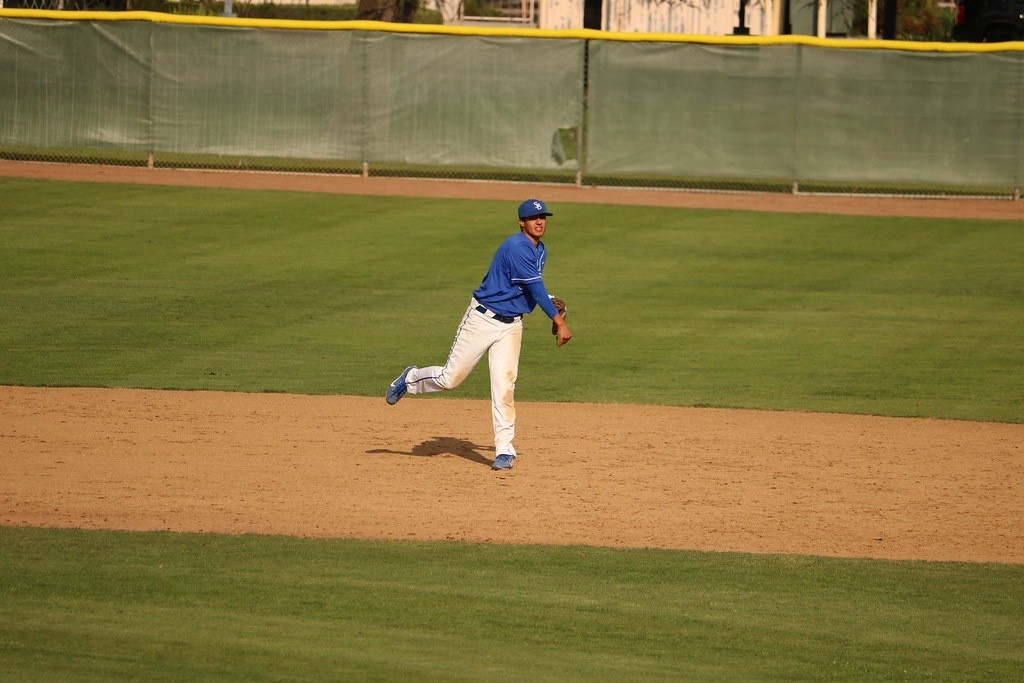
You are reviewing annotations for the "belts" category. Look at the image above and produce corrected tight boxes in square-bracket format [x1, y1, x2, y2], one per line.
[477, 306, 523, 324]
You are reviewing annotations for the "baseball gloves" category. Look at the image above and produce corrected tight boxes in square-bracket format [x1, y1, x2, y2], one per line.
[548, 293, 567, 335]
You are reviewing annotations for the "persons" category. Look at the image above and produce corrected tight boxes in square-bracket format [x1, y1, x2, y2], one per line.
[382, 197, 572, 471]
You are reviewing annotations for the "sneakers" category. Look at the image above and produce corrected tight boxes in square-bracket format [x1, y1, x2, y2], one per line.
[386, 365, 418, 405]
[491, 454, 516, 469]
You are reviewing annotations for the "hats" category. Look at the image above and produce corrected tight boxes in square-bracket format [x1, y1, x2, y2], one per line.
[518, 199, 553, 219]
